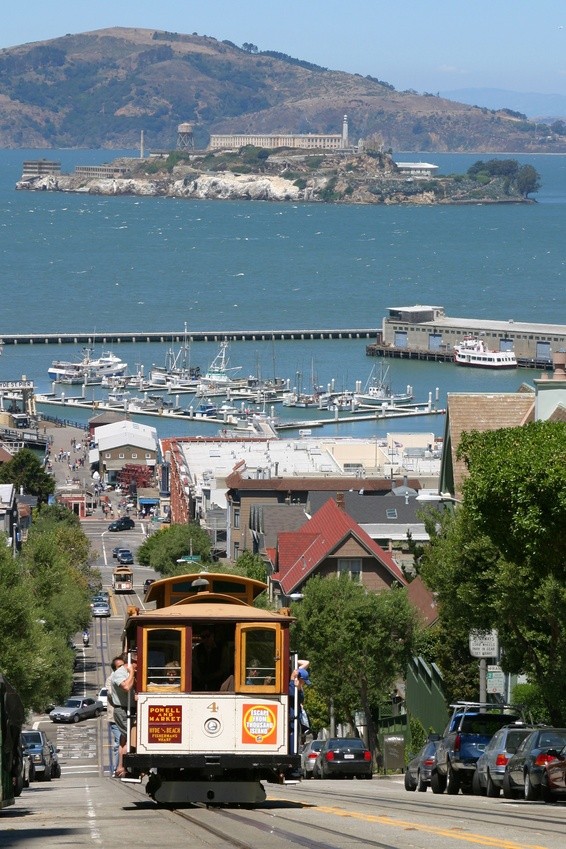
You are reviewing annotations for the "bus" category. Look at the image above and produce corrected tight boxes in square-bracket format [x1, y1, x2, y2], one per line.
[111, 566, 134, 594]
[118, 573, 306, 809]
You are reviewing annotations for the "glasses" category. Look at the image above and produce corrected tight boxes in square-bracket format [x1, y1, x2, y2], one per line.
[201, 632, 212, 638]
[252, 669, 260, 672]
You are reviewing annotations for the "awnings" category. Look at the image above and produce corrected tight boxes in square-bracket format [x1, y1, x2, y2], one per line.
[89, 448, 99, 464]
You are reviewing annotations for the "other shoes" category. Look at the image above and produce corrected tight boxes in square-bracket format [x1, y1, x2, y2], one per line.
[115, 768, 136, 778]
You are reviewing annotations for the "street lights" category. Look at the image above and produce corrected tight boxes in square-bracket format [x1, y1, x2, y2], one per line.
[374, 411, 379, 468]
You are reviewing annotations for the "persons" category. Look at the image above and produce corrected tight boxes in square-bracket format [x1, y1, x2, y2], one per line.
[105, 656, 125, 776]
[94, 484, 101, 496]
[288, 660, 310, 754]
[139, 508, 145, 519]
[35, 425, 39, 433]
[68, 454, 85, 472]
[110, 652, 138, 777]
[150, 507, 156, 517]
[71, 437, 88, 453]
[161, 661, 180, 684]
[118, 508, 121, 518]
[44, 424, 46, 434]
[102, 502, 113, 520]
[82, 628, 90, 637]
[55, 448, 70, 462]
[246, 659, 272, 685]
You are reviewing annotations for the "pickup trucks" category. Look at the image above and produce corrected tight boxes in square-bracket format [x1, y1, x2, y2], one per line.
[428, 701, 525, 795]
[143, 578, 156, 593]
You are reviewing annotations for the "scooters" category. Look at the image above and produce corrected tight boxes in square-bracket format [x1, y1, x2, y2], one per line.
[83, 634, 88, 647]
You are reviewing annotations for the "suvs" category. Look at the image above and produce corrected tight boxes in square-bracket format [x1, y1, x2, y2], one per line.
[0, 673, 61, 809]
[108, 517, 135, 532]
[472, 724, 556, 797]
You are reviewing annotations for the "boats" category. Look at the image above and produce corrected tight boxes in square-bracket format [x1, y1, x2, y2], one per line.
[126, 322, 358, 411]
[101, 375, 125, 389]
[100, 381, 138, 405]
[355, 356, 413, 405]
[48, 326, 127, 383]
[453, 336, 517, 370]
[128, 392, 266, 420]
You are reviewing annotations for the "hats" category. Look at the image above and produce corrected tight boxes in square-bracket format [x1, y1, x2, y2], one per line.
[299, 669, 312, 686]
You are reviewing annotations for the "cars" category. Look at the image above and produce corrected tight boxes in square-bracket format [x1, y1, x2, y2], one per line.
[88, 578, 112, 617]
[49, 697, 103, 724]
[299, 738, 372, 780]
[96, 687, 109, 709]
[405, 741, 441, 792]
[504, 728, 566, 801]
[111, 547, 133, 564]
